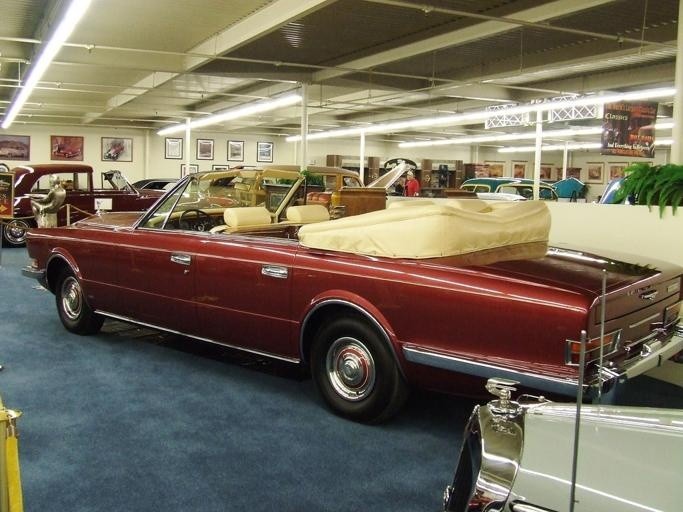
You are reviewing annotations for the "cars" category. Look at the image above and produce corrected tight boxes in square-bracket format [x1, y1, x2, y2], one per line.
[598, 175, 639, 205]
[0, 164, 170, 246]
[126, 177, 179, 190]
[22, 164, 683, 424]
[0, 143, 27, 158]
[207, 164, 411, 212]
[458, 177, 588, 203]
[53, 143, 80, 158]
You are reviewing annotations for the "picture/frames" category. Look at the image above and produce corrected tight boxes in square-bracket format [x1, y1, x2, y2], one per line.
[164, 137, 183, 159]
[49, 134, 84, 162]
[211, 164, 228, 171]
[256, 141, 273, 163]
[180, 163, 198, 178]
[0, 134, 31, 162]
[482, 160, 653, 185]
[226, 140, 244, 162]
[100, 137, 133, 162]
[195, 138, 213, 161]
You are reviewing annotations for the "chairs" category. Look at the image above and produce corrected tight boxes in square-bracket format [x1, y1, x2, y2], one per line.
[279, 205, 331, 224]
[208, 206, 272, 234]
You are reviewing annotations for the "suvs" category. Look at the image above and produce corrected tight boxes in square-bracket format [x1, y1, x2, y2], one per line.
[441, 378, 682, 511]
[103, 140, 124, 160]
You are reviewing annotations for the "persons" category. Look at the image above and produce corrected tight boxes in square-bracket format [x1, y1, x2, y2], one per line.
[404, 172, 423, 197]
[27, 172, 69, 221]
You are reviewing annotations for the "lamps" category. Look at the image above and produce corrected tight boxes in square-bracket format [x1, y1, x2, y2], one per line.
[0, 0, 94, 129]
